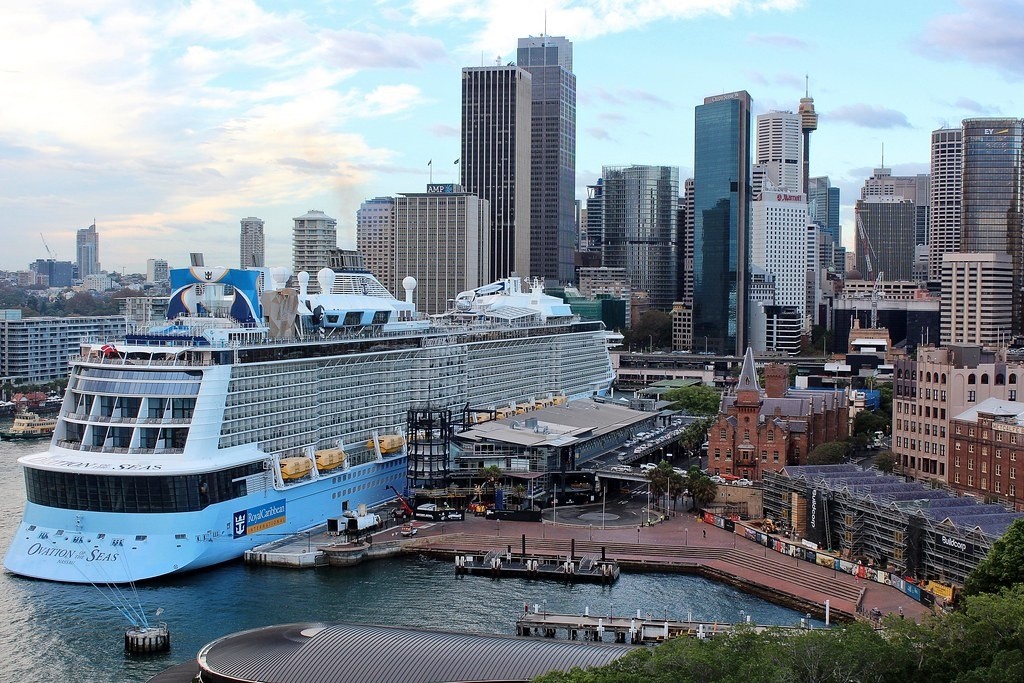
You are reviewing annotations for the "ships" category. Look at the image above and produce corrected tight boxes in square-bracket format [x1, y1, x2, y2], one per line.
[4, 273, 616, 583]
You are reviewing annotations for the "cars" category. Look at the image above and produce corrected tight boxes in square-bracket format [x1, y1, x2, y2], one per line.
[610, 417, 753, 487]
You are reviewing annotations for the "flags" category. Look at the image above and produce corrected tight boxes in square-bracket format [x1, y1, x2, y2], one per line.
[101, 342, 117, 355]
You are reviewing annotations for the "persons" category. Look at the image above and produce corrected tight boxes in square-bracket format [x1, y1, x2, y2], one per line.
[702, 528, 706, 538]
[855, 573, 859, 584]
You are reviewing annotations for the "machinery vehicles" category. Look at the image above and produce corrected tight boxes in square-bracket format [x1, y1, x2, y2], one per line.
[383, 485, 414, 518]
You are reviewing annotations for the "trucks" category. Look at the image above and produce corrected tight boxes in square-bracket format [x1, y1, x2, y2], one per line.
[400, 523, 417, 537]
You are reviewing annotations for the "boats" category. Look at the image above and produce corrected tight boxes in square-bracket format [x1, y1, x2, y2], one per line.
[0, 407, 59, 439]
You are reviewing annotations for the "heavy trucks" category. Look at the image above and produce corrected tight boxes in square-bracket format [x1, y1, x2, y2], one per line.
[347, 512, 382, 535]
